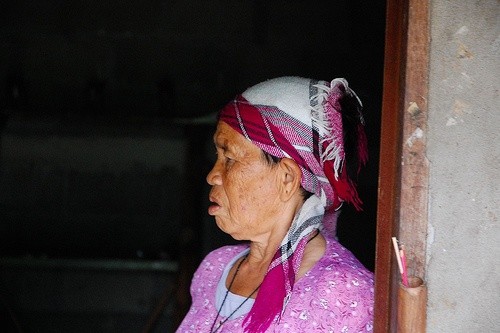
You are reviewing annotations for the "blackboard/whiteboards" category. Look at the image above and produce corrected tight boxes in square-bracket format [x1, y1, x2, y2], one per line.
[1, 0, 388, 270]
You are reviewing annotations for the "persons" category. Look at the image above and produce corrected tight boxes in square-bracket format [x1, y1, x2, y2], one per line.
[174, 75, 375, 333]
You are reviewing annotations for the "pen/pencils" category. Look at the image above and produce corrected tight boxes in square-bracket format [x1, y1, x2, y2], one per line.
[391, 236, 409, 286]
